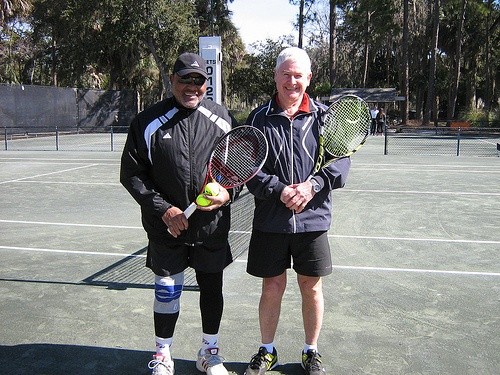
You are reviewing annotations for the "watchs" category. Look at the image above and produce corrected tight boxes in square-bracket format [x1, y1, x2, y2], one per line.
[309, 179, 321, 192]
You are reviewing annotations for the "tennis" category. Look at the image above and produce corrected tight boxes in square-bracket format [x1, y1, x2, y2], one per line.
[205, 183, 220, 196]
[196, 194, 212, 207]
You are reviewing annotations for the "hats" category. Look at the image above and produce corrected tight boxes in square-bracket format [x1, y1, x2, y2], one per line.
[173, 53, 209, 80]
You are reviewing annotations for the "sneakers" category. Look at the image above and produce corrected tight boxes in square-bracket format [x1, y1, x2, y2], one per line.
[242, 346, 279, 375]
[196, 348, 229, 375]
[301, 348, 327, 375]
[147, 354, 176, 375]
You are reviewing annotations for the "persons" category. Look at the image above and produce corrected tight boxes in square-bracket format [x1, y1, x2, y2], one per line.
[120, 53, 244, 375]
[370, 105, 386, 136]
[242, 47, 351, 375]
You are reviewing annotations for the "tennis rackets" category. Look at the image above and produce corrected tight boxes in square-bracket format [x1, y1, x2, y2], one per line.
[167, 126, 269, 237]
[304, 94, 372, 182]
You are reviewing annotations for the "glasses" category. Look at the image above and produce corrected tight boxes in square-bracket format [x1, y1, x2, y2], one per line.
[177, 73, 207, 86]
